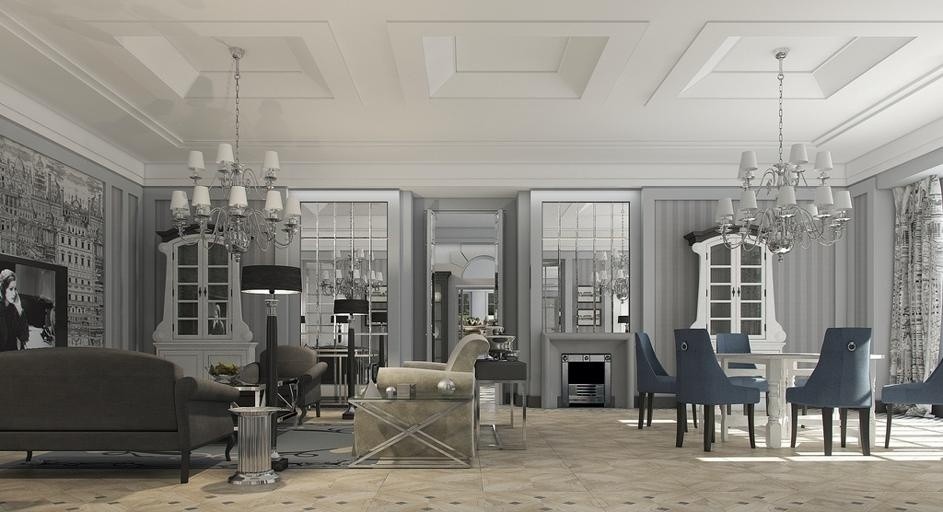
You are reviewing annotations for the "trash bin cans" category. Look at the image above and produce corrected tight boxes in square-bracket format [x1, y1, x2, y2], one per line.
[227, 407, 292, 486]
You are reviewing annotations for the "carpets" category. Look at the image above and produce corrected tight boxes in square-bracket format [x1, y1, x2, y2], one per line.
[0, 422, 379, 469]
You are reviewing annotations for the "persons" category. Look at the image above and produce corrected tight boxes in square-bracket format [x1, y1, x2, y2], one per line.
[0, 267, 30, 352]
[206, 304, 224, 335]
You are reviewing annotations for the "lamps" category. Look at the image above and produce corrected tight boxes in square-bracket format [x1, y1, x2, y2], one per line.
[240, 265, 302, 472]
[320, 248, 387, 303]
[168, 47, 300, 263]
[333, 299, 368, 420]
[593, 202, 629, 304]
[330, 311, 386, 402]
[714, 48, 853, 264]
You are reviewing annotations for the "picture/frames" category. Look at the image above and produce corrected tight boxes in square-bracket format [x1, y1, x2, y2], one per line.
[577, 284, 601, 326]
[364, 284, 387, 326]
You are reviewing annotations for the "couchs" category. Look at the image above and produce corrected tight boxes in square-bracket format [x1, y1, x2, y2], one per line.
[0, 346, 241, 483]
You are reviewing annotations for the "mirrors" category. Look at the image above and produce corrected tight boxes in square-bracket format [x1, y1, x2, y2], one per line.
[540, 201, 629, 334]
[298, 201, 388, 406]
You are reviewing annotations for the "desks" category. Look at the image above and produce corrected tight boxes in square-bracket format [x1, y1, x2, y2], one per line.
[475, 358, 527, 452]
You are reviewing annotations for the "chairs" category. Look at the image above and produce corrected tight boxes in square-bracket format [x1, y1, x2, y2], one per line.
[352, 333, 490, 461]
[240, 344, 328, 426]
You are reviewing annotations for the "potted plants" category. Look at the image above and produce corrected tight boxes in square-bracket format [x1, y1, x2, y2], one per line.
[208, 361, 239, 384]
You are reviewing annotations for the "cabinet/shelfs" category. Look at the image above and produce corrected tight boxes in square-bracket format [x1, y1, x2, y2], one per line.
[682, 223, 787, 411]
[151, 223, 258, 381]
[432, 271, 450, 362]
